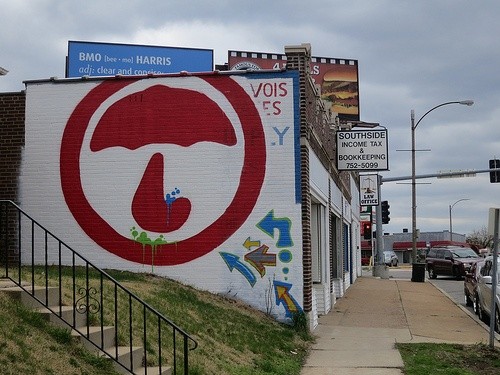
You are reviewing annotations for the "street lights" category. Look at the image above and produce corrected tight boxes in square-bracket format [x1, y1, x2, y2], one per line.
[411, 100, 474, 263]
[449, 198, 470, 241]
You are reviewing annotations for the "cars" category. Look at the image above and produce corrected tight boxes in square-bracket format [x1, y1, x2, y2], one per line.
[462, 248, 500, 332]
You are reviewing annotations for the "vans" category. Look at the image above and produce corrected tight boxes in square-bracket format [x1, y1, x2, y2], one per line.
[374, 251, 399, 267]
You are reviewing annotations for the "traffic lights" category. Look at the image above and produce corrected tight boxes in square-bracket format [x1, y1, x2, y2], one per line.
[364, 224, 371, 239]
[381, 200, 391, 225]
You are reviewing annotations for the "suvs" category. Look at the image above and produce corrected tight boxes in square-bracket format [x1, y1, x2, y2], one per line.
[425, 245, 480, 281]
